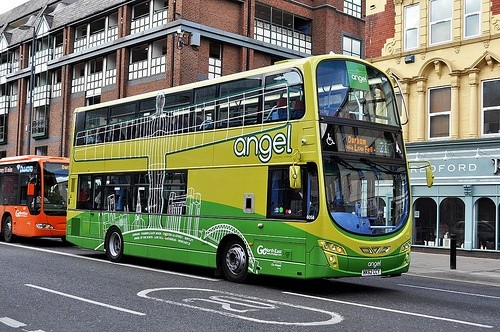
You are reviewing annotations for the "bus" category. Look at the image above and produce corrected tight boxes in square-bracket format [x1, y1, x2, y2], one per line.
[0, 155, 70, 243]
[64, 51, 436, 284]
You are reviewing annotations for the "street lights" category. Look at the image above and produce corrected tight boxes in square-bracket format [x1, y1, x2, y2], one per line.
[18, 24, 35, 155]
